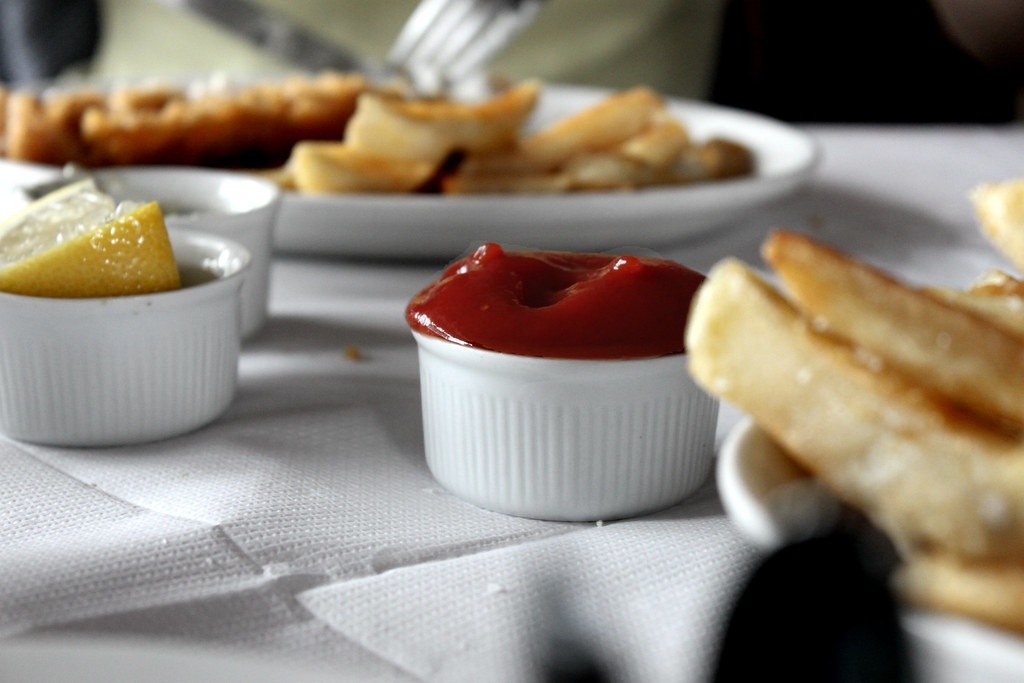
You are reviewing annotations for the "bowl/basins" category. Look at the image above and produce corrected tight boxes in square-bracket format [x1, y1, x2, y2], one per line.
[412, 328, 721, 522]
[0, 228, 253, 448]
[19, 167, 281, 342]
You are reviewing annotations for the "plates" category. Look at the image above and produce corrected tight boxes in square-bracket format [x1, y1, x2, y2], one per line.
[715, 416, 1024, 683]
[0, 87, 822, 260]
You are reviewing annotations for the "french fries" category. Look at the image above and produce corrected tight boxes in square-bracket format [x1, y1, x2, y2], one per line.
[279, 84, 752, 198]
[680, 178, 1024, 635]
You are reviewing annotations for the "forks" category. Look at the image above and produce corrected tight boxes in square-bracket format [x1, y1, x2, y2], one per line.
[386, 0, 540, 86]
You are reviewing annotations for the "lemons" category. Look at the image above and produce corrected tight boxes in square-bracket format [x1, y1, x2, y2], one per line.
[0, 178, 182, 297]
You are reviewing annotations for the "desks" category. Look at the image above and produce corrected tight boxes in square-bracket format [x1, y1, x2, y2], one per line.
[0, 125, 1024, 683]
